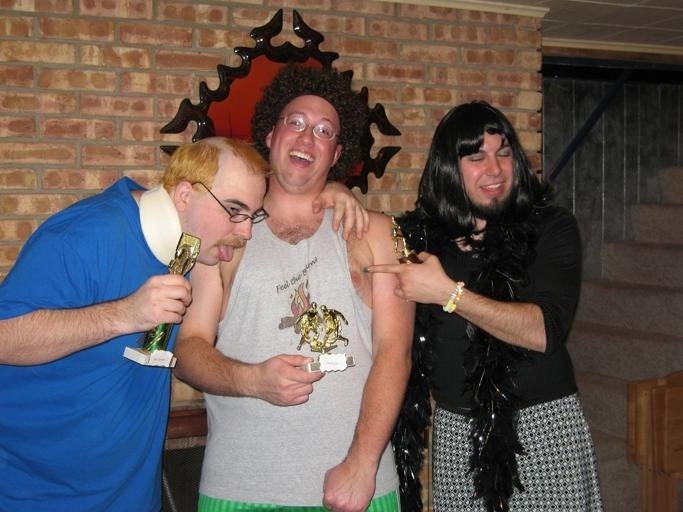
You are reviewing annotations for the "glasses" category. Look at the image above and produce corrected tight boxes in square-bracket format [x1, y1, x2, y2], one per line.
[189, 182, 269, 224]
[277, 115, 344, 141]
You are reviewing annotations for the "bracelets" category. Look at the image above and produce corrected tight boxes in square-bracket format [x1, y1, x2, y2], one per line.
[443, 279, 467, 315]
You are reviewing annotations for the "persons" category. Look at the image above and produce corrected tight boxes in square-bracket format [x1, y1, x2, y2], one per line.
[169, 88, 416, 512]
[0, 135, 370, 511]
[361, 99, 606, 511]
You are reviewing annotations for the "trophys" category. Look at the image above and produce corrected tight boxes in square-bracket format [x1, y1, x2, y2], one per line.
[294, 300, 356, 373]
[389, 213, 429, 264]
[119, 231, 202, 371]
